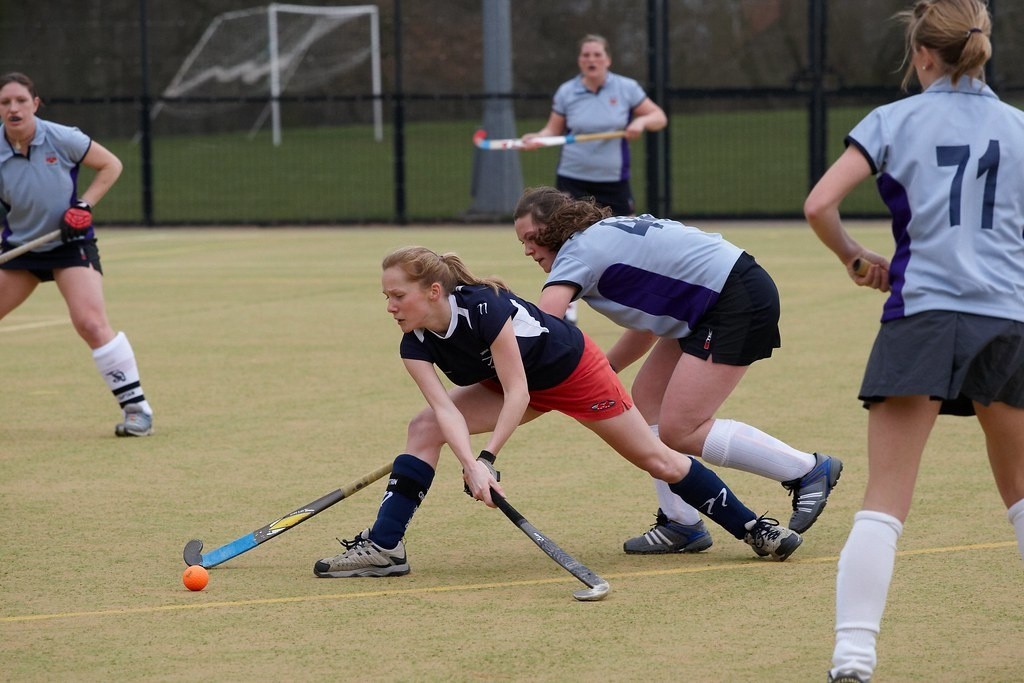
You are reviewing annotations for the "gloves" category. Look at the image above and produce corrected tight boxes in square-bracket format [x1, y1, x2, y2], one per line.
[59, 202, 92, 246]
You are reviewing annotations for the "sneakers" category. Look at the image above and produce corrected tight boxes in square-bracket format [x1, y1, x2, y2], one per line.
[744, 510, 803, 562]
[781, 452, 843, 534]
[622, 507, 713, 555]
[313, 528, 410, 578]
[115, 404, 154, 437]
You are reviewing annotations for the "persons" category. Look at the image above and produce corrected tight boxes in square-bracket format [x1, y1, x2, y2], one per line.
[314, 246, 803, 579]
[514, 188, 843, 555]
[0, 69, 154, 437]
[803, 0, 1024, 683]
[521, 32, 668, 324]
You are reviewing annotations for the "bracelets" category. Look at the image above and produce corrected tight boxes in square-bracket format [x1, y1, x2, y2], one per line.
[477, 450, 496, 464]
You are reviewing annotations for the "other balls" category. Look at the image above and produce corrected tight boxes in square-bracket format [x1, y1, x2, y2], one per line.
[184, 566, 208, 591]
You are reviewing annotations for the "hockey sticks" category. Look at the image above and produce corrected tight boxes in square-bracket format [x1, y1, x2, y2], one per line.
[462, 470, 609, 601]
[851, 257, 883, 280]
[0, 230, 62, 264]
[183, 460, 395, 567]
[473, 130, 626, 150]
